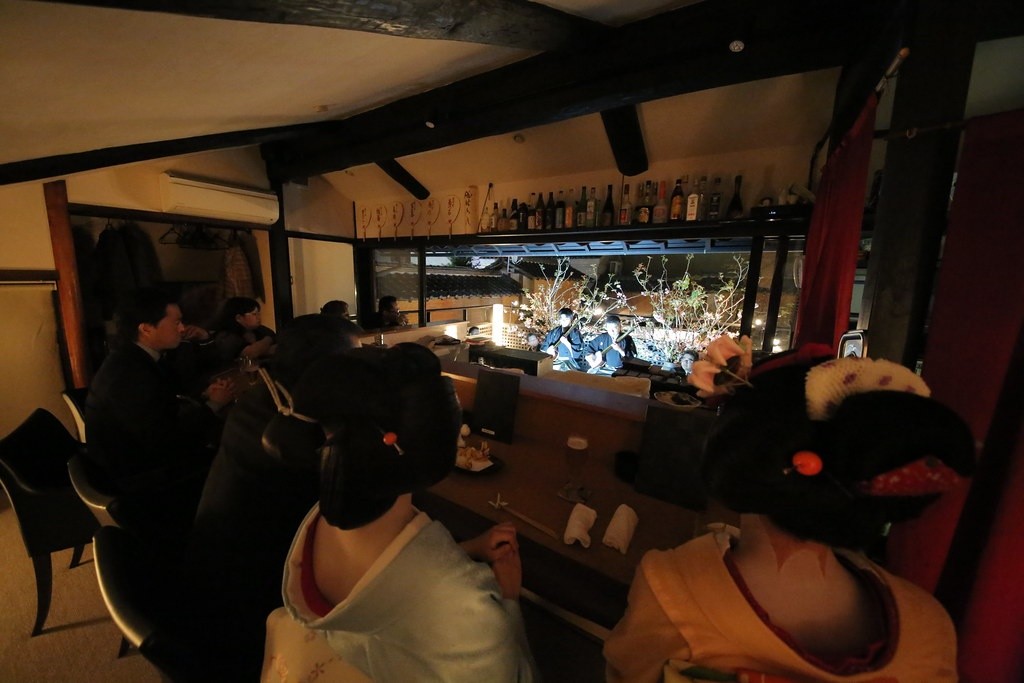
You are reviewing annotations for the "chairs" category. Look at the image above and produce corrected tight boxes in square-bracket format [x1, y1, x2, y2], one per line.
[0, 387, 255, 683]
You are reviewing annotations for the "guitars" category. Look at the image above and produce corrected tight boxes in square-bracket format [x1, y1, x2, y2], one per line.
[547, 317, 587, 362]
[585, 325, 635, 369]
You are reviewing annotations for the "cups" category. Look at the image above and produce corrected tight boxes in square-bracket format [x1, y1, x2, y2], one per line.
[566, 433, 590, 498]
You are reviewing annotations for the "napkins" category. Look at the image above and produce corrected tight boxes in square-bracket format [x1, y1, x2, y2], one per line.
[564, 503, 639, 555]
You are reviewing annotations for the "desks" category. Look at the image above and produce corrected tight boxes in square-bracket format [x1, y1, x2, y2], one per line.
[468, 345, 553, 376]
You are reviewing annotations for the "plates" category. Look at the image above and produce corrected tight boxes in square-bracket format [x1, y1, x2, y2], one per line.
[653, 390, 702, 410]
[453, 454, 507, 476]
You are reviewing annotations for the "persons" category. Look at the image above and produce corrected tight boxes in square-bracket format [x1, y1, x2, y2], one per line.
[469, 326, 479, 335]
[281, 343, 541, 683]
[74, 288, 364, 613]
[601, 343, 978, 683]
[361, 295, 408, 329]
[527, 333, 541, 352]
[585, 315, 637, 375]
[542, 308, 583, 371]
[670, 350, 699, 375]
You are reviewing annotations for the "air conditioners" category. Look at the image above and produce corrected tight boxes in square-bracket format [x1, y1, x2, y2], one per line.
[160, 173, 279, 225]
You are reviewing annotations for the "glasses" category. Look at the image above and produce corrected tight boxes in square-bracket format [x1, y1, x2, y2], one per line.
[244, 312, 261, 316]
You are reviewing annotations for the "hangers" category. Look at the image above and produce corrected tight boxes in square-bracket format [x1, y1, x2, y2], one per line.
[158, 224, 249, 251]
[99, 217, 148, 237]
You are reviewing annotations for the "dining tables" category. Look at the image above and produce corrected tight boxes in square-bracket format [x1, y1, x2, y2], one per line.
[194, 348, 743, 683]
